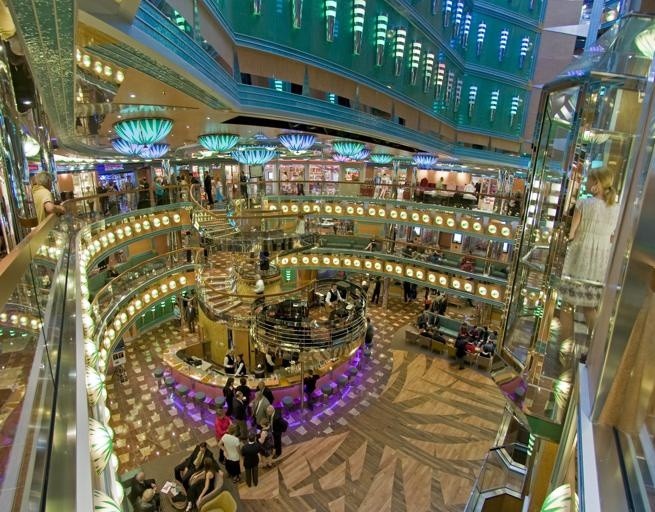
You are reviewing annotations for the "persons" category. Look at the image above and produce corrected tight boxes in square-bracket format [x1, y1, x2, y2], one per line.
[462, 178, 499, 195]
[32, 171, 66, 224]
[107, 265, 128, 292]
[303, 369, 320, 411]
[556, 166, 621, 364]
[371, 172, 393, 201]
[304, 217, 353, 236]
[296, 216, 311, 247]
[325, 276, 499, 371]
[250, 272, 266, 311]
[365, 239, 379, 252]
[182, 231, 194, 263]
[173, 287, 198, 334]
[399, 243, 445, 266]
[434, 177, 445, 191]
[458, 249, 475, 274]
[215, 348, 297, 487]
[126, 440, 225, 512]
[83, 170, 306, 218]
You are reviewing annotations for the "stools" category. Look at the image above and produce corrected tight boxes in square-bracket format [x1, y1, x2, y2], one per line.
[153, 340, 373, 414]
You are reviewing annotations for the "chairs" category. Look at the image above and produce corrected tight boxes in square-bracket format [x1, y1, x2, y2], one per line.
[405, 311, 497, 370]
[119, 443, 236, 511]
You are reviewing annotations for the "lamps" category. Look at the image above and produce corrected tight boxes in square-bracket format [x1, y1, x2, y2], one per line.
[370, 154, 394, 165]
[351, 151, 371, 162]
[276, 132, 318, 156]
[110, 138, 152, 156]
[330, 140, 365, 157]
[196, 133, 240, 153]
[329, 153, 352, 165]
[137, 144, 169, 159]
[111, 118, 175, 142]
[411, 153, 439, 169]
[231, 147, 277, 167]
